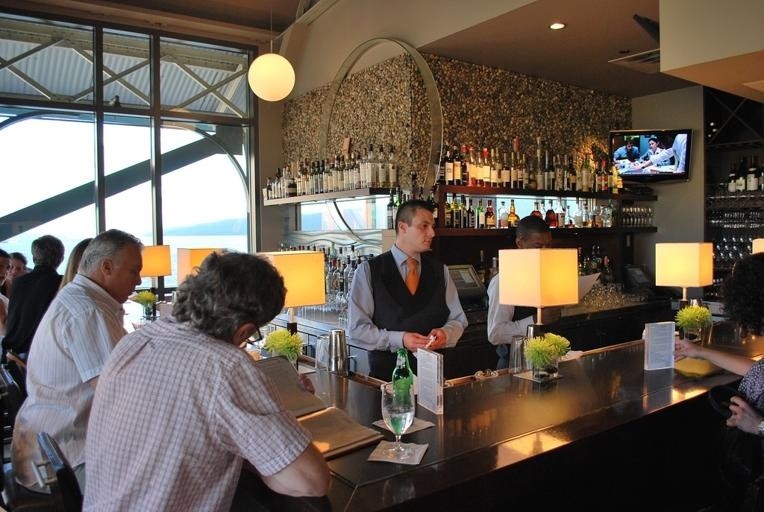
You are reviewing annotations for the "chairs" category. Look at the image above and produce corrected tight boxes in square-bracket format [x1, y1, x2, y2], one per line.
[0, 427, 59, 512]
[0, 364, 23, 429]
[29, 431, 83, 512]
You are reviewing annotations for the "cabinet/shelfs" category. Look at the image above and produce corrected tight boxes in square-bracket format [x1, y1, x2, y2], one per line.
[703, 192, 764, 302]
[263, 183, 396, 330]
[437, 185, 660, 236]
[435, 296, 675, 380]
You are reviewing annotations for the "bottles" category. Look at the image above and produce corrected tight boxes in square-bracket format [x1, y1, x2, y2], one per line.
[477, 201, 485, 228]
[527, 154, 534, 181]
[608, 161, 616, 188]
[483, 148, 491, 188]
[589, 169, 593, 192]
[523, 153, 530, 189]
[444, 194, 451, 227]
[476, 152, 483, 187]
[583, 203, 588, 226]
[554, 197, 565, 228]
[485, 200, 496, 229]
[477, 250, 496, 283]
[498, 201, 509, 228]
[602, 159, 608, 192]
[262, 143, 397, 199]
[540, 199, 546, 221]
[490, 148, 496, 187]
[531, 202, 542, 219]
[567, 155, 576, 191]
[437, 156, 445, 185]
[545, 149, 550, 191]
[555, 154, 564, 191]
[460, 144, 469, 185]
[621, 207, 651, 224]
[564, 155, 568, 191]
[573, 203, 582, 226]
[453, 144, 463, 185]
[387, 185, 406, 229]
[428, 191, 438, 225]
[451, 198, 460, 228]
[582, 160, 590, 192]
[466, 199, 475, 228]
[325, 241, 362, 299]
[501, 153, 510, 188]
[726, 153, 764, 192]
[459, 195, 466, 228]
[593, 160, 602, 193]
[392, 348, 413, 407]
[545, 198, 556, 228]
[507, 199, 520, 228]
[577, 244, 612, 285]
[550, 160, 556, 190]
[495, 146, 502, 188]
[444, 148, 453, 185]
[589, 205, 599, 227]
[565, 206, 574, 228]
[510, 151, 517, 189]
[517, 152, 522, 189]
[468, 147, 477, 186]
[535, 145, 545, 190]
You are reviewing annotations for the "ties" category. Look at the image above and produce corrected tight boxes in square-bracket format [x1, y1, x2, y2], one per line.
[402, 258, 420, 296]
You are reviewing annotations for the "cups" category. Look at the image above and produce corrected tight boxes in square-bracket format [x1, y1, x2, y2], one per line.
[382, 383, 416, 462]
[316, 335, 330, 373]
[508, 336, 529, 374]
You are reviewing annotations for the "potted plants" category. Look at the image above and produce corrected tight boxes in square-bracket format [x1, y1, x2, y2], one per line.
[263, 331, 302, 366]
[674, 305, 712, 343]
[527, 333, 571, 380]
[134, 290, 157, 318]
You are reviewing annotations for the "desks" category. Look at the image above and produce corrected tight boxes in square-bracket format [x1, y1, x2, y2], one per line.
[243, 324, 764, 511]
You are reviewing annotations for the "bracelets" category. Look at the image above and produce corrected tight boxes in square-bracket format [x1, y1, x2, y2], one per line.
[759, 420, 764, 435]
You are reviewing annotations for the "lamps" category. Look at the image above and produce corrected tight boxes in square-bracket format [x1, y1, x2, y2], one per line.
[247, 52, 295, 103]
[654, 242, 714, 311]
[498, 247, 578, 336]
[141, 246, 171, 294]
[177, 247, 224, 282]
[258, 250, 325, 333]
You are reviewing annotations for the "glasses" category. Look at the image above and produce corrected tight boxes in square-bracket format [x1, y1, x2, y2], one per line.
[243, 329, 264, 345]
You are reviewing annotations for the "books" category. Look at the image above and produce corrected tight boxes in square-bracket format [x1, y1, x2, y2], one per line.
[255, 356, 384, 461]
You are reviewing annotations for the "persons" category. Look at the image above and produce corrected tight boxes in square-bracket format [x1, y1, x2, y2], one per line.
[613, 140, 639, 163]
[2, 236, 64, 361]
[57, 238, 94, 291]
[82, 252, 332, 511]
[488, 216, 562, 371]
[639, 138, 670, 166]
[348, 199, 469, 382]
[0, 250, 12, 337]
[674, 253, 764, 434]
[5, 253, 30, 297]
[10, 229, 143, 495]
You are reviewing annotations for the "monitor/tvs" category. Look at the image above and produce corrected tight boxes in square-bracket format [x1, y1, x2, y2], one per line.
[608, 128, 693, 185]
[447, 265, 486, 297]
